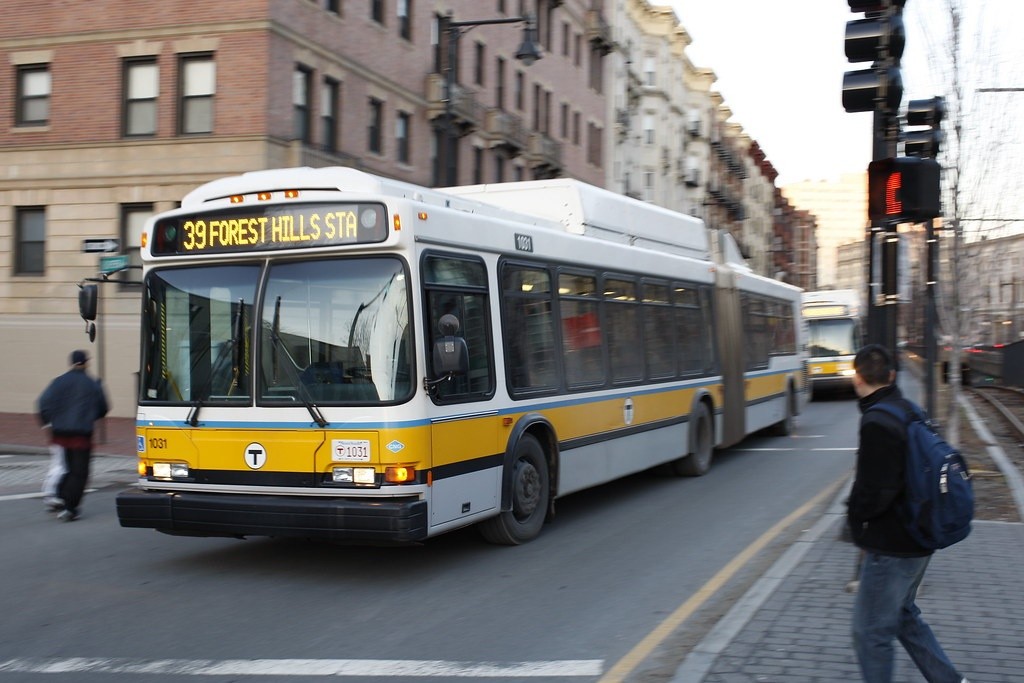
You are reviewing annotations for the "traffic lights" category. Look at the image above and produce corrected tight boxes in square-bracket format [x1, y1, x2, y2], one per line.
[844, 1, 902, 112]
[865, 156, 943, 226]
[897, 94, 947, 156]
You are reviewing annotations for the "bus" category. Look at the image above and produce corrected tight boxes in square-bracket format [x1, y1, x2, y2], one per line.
[798, 287, 867, 399]
[74, 163, 813, 547]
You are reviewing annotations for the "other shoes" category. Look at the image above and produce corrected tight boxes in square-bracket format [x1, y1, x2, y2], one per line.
[58, 510, 79, 520]
[47, 497, 66, 510]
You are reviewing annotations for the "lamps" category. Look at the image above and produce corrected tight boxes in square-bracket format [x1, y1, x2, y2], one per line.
[440, 15, 542, 110]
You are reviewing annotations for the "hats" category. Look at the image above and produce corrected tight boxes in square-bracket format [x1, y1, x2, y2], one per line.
[71, 351, 90, 365]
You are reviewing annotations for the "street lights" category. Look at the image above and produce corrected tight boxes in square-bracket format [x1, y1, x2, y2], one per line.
[442, 14, 546, 188]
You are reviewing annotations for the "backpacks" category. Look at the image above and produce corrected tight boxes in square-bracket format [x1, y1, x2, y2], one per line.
[864, 402, 975, 549]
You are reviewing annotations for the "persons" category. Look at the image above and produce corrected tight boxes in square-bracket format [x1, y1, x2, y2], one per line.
[838, 343, 973, 682]
[33, 350, 109, 519]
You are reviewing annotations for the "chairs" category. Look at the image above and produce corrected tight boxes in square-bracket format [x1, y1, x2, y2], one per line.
[431, 337, 470, 395]
